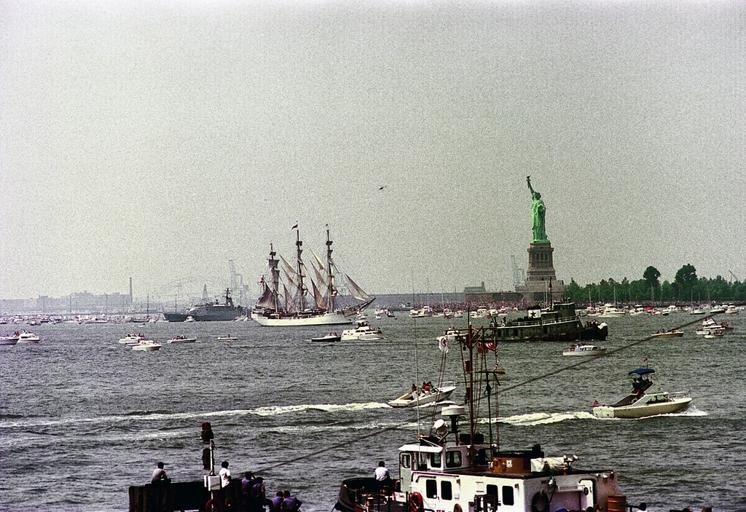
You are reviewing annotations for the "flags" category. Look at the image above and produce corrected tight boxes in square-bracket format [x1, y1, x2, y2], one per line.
[486, 343, 496, 351]
[439, 337, 450, 355]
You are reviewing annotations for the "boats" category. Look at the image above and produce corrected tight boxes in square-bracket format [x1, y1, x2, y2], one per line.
[447, 277, 608, 340]
[119, 332, 162, 352]
[341, 325, 383, 341]
[374, 308, 395, 320]
[389, 379, 456, 407]
[312, 332, 341, 341]
[0, 329, 40, 345]
[604, 301, 740, 316]
[696, 314, 730, 337]
[628, 357, 655, 395]
[167, 335, 197, 344]
[593, 389, 693, 420]
[652, 328, 684, 338]
[469, 306, 510, 318]
[0, 313, 159, 325]
[217, 333, 238, 341]
[564, 345, 604, 356]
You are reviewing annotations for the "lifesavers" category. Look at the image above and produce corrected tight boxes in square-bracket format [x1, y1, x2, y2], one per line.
[409, 492, 423, 511]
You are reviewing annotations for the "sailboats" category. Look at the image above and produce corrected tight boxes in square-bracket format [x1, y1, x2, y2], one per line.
[409, 279, 463, 318]
[332, 321, 646, 512]
[355, 315, 368, 327]
[251, 220, 376, 326]
[190, 284, 248, 321]
[163, 293, 189, 322]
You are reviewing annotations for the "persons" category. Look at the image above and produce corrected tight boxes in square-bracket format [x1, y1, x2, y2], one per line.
[411, 379, 435, 399]
[221, 461, 232, 506]
[526, 174, 550, 244]
[373, 460, 391, 487]
[242, 472, 302, 512]
[633, 378, 638, 387]
[150, 463, 172, 484]
